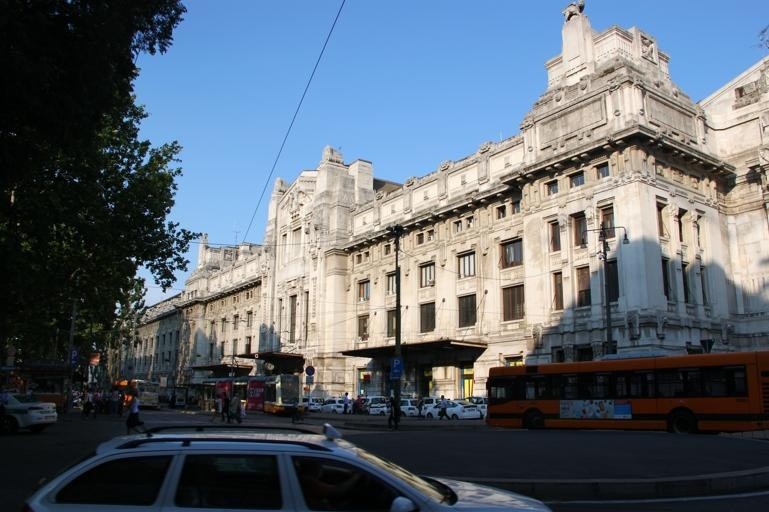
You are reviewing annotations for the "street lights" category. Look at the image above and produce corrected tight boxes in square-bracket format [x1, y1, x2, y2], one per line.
[580, 222, 630, 354]
[385, 224, 407, 420]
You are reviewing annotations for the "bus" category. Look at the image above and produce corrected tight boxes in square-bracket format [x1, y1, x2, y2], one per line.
[486, 348, 769, 435]
[200, 375, 304, 416]
[111, 379, 160, 410]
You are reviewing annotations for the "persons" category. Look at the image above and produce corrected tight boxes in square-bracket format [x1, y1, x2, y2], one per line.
[342, 392, 364, 415]
[121, 387, 142, 435]
[208, 391, 243, 424]
[387, 397, 400, 430]
[292, 397, 306, 424]
[415, 397, 426, 421]
[433, 395, 451, 420]
[82, 395, 101, 421]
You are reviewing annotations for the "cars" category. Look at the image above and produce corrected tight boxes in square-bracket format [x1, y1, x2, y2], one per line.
[23, 422, 556, 512]
[304, 396, 488, 420]
[1, 392, 58, 433]
